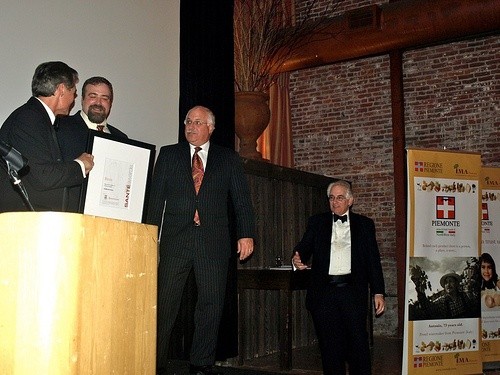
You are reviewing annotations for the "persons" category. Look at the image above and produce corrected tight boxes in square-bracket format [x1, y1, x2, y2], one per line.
[480, 252, 500, 293]
[291, 179, 385, 375]
[145, 106, 255, 375]
[410, 272, 478, 319]
[0, 60, 128, 213]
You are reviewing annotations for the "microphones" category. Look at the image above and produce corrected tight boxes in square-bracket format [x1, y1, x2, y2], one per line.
[0, 140, 30, 169]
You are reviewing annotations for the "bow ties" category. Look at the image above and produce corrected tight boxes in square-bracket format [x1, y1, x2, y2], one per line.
[334, 213, 347, 223]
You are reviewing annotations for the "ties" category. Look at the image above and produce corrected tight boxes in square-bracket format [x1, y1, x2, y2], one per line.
[97, 125, 105, 133]
[191, 146, 201, 226]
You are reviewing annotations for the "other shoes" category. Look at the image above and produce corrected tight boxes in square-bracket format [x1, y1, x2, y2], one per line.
[187, 362, 225, 375]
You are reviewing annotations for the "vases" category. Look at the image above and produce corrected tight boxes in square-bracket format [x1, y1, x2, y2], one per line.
[234, 91, 270, 161]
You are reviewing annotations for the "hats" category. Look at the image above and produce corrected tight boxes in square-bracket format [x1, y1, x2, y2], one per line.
[440, 269, 462, 288]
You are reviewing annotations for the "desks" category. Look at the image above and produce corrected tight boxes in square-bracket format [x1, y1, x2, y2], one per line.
[239, 267, 374, 370]
